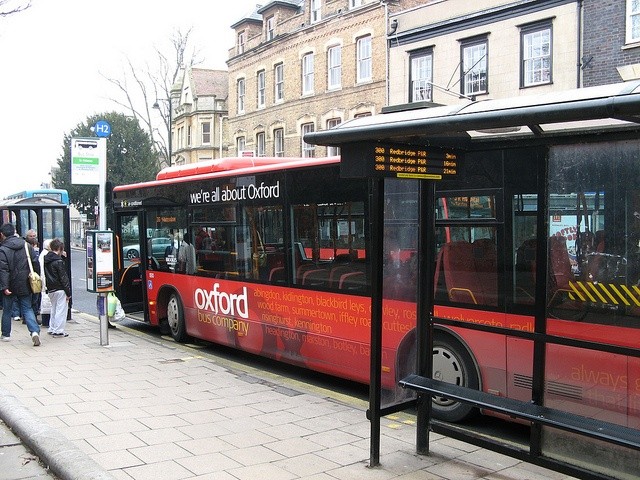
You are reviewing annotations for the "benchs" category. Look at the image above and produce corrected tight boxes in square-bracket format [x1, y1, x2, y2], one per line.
[399, 373, 639, 450]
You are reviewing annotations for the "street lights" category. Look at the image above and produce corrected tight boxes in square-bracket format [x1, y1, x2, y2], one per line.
[152, 98, 172, 167]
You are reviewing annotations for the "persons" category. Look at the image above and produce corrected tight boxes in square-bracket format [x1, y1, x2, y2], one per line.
[195, 222, 226, 280]
[11, 299, 21, 321]
[44, 239, 72, 338]
[22, 230, 42, 324]
[0, 224, 41, 347]
[107, 226, 116, 328]
[38, 239, 55, 328]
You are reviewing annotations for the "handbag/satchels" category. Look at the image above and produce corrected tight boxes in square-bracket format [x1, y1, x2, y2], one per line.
[108, 291, 116, 317]
[108, 295, 126, 326]
[25, 241, 42, 293]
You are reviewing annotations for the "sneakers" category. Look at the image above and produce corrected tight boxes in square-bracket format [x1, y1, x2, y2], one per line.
[52, 333, 69, 338]
[0, 335, 9, 342]
[31, 332, 41, 346]
[13, 316, 21, 321]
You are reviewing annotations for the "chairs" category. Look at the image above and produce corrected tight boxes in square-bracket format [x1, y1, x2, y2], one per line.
[473, 238, 535, 305]
[339, 271, 366, 290]
[442, 240, 484, 305]
[548, 236, 588, 311]
[329, 266, 351, 288]
[296, 263, 312, 285]
[268, 267, 284, 283]
[302, 269, 329, 289]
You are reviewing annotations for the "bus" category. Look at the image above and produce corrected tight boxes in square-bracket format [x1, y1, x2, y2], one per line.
[4, 188, 71, 255]
[110, 133, 640, 459]
